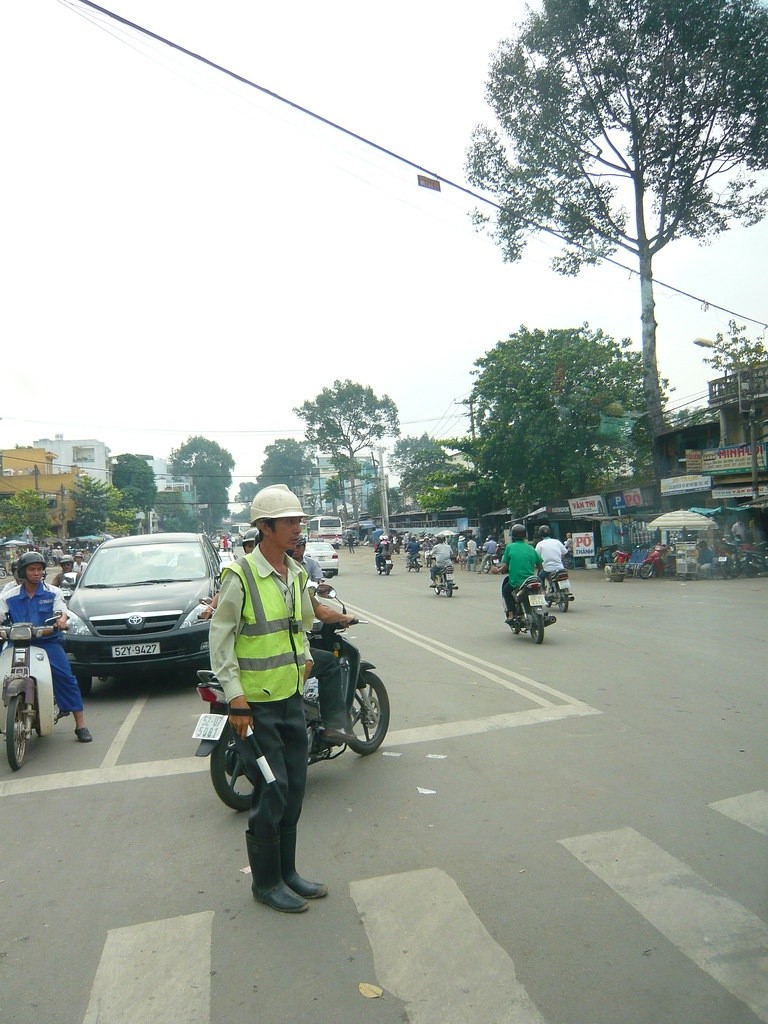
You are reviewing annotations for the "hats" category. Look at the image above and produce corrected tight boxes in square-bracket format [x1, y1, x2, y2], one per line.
[424, 537, 429, 539]
[458, 535, 466, 541]
[296, 535, 306, 546]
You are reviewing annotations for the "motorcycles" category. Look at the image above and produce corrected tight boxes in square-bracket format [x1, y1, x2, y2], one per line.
[425, 556, 459, 597]
[190, 585, 390, 812]
[535, 549, 575, 614]
[486, 564, 557, 644]
[374, 534, 502, 576]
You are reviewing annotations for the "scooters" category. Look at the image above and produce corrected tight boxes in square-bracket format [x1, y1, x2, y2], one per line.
[0, 552, 81, 605]
[560, 534, 768, 579]
[0, 609, 71, 772]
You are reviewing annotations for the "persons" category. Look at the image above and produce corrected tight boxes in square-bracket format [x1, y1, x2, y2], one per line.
[348, 534, 355, 553]
[564, 532, 573, 556]
[491, 524, 544, 623]
[456, 534, 496, 574]
[373, 531, 432, 571]
[0, 552, 93, 742]
[209, 484, 328, 913]
[535, 525, 568, 592]
[731, 519, 746, 543]
[201, 527, 357, 743]
[695, 541, 713, 575]
[429, 534, 454, 587]
[52, 546, 63, 566]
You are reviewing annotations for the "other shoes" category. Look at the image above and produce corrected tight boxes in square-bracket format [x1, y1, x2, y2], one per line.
[430, 584, 436, 587]
[477, 571, 482, 574]
[505, 618, 513, 623]
[75, 727, 92, 742]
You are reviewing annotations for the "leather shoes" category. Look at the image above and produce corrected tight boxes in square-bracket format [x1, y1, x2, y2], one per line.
[320, 729, 357, 744]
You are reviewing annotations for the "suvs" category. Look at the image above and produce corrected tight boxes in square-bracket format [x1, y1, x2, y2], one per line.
[59, 533, 222, 694]
[342, 530, 360, 546]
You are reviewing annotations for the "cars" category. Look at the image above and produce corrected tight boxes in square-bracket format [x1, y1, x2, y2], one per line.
[166, 550, 239, 568]
[303, 542, 339, 575]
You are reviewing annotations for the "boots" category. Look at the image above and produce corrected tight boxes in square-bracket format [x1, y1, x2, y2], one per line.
[246, 830, 310, 913]
[280, 827, 328, 899]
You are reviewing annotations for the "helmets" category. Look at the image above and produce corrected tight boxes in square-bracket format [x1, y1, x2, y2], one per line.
[17, 551, 46, 575]
[539, 525, 551, 535]
[242, 527, 260, 542]
[383, 535, 389, 539]
[488, 535, 494, 539]
[59, 555, 74, 563]
[412, 536, 416, 540]
[251, 484, 308, 526]
[436, 534, 445, 543]
[11, 558, 20, 571]
[379, 535, 383, 540]
[75, 551, 85, 558]
[511, 524, 526, 539]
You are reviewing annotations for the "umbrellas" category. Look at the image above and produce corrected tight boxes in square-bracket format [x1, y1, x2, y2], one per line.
[647, 509, 718, 541]
[435, 530, 459, 537]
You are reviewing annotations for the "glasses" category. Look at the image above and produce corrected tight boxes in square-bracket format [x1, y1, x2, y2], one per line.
[62, 563, 70, 565]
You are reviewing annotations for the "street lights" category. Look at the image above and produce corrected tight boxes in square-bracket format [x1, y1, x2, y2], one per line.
[692, 335, 759, 500]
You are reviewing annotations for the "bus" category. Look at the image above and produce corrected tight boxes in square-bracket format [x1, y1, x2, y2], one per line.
[307, 515, 343, 548]
[230, 522, 251, 546]
